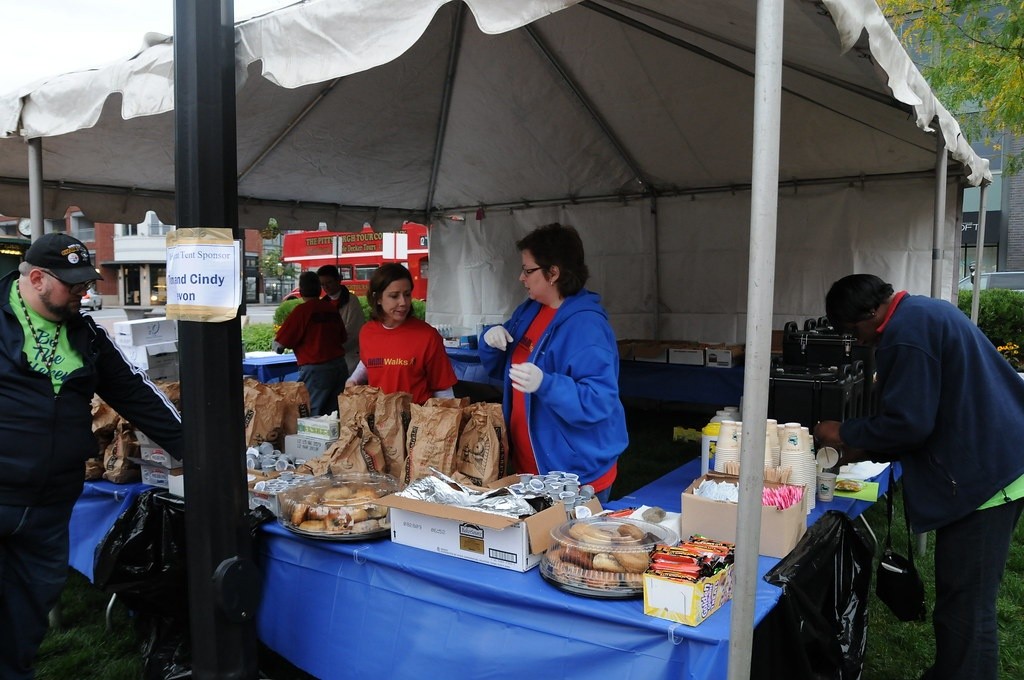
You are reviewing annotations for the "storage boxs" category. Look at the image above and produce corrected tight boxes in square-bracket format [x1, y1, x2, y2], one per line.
[443, 335, 477, 350]
[616, 339, 746, 367]
[126, 415, 807, 626]
[114, 315, 178, 378]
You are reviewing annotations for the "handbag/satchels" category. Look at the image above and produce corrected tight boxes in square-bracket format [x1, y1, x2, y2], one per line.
[876, 551, 927, 622]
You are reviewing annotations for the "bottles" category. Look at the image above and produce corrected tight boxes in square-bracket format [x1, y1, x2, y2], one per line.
[432, 324, 452, 337]
[701, 423, 720, 476]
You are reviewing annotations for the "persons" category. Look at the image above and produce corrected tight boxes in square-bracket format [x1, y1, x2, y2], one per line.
[814, 274, 1024, 680]
[317, 265, 366, 376]
[0, 232, 183, 679]
[274, 271, 349, 417]
[346, 262, 458, 402]
[479, 223, 628, 503]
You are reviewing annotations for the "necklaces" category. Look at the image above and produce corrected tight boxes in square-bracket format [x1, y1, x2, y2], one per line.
[17, 277, 63, 381]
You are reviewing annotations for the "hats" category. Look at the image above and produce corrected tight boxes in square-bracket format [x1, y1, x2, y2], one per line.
[25, 232, 104, 283]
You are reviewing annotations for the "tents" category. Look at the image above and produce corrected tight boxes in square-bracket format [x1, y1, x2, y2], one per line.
[0, 2, 991, 680]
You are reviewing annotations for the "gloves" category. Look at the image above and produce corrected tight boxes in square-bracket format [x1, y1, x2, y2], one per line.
[484, 325, 514, 351]
[509, 362, 543, 394]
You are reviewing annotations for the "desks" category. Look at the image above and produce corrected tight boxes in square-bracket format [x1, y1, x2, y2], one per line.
[69, 446, 927, 679]
[242, 346, 744, 408]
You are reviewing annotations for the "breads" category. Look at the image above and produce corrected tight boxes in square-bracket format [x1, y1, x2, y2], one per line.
[835, 480, 860, 491]
[545, 524, 652, 587]
[290, 482, 393, 534]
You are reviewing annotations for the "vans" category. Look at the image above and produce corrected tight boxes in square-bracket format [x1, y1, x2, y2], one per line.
[958, 272, 1024, 291]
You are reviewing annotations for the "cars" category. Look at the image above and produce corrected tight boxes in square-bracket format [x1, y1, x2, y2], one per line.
[80, 287, 102, 310]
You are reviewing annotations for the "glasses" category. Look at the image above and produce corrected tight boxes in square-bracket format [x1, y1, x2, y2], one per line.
[40, 269, 93, 295]
[521, 265, 553, 277]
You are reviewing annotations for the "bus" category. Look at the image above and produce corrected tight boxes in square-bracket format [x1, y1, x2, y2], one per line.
[283, 222, 429, 302]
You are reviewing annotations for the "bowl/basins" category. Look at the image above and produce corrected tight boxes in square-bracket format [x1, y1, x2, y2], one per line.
[710, 407, 739, 422]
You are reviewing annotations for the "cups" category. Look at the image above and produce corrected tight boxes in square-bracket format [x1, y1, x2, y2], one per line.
[818, 472, 837, 501]
[715, 419, 817, 515]
[816, 446, 839, 469]
